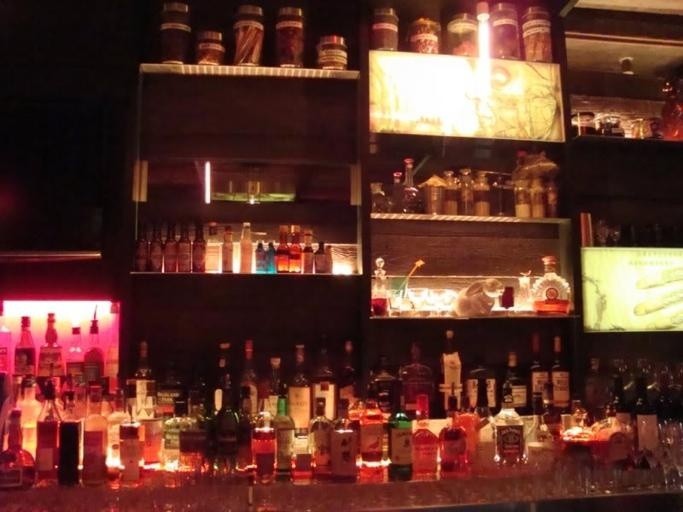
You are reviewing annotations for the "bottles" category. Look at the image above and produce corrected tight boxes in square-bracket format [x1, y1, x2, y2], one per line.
[369, 146, 560, 219]
[572, 80, 683, 141]
[0, 312, 683, 490]
[371, 257, 390, 318]
[157, 2, 554, 70]
[133, 216, 333, 274]
[529, 256, 572, 317]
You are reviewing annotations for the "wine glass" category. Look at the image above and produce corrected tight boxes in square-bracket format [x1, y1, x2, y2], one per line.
[594, 214, 623, 247]
